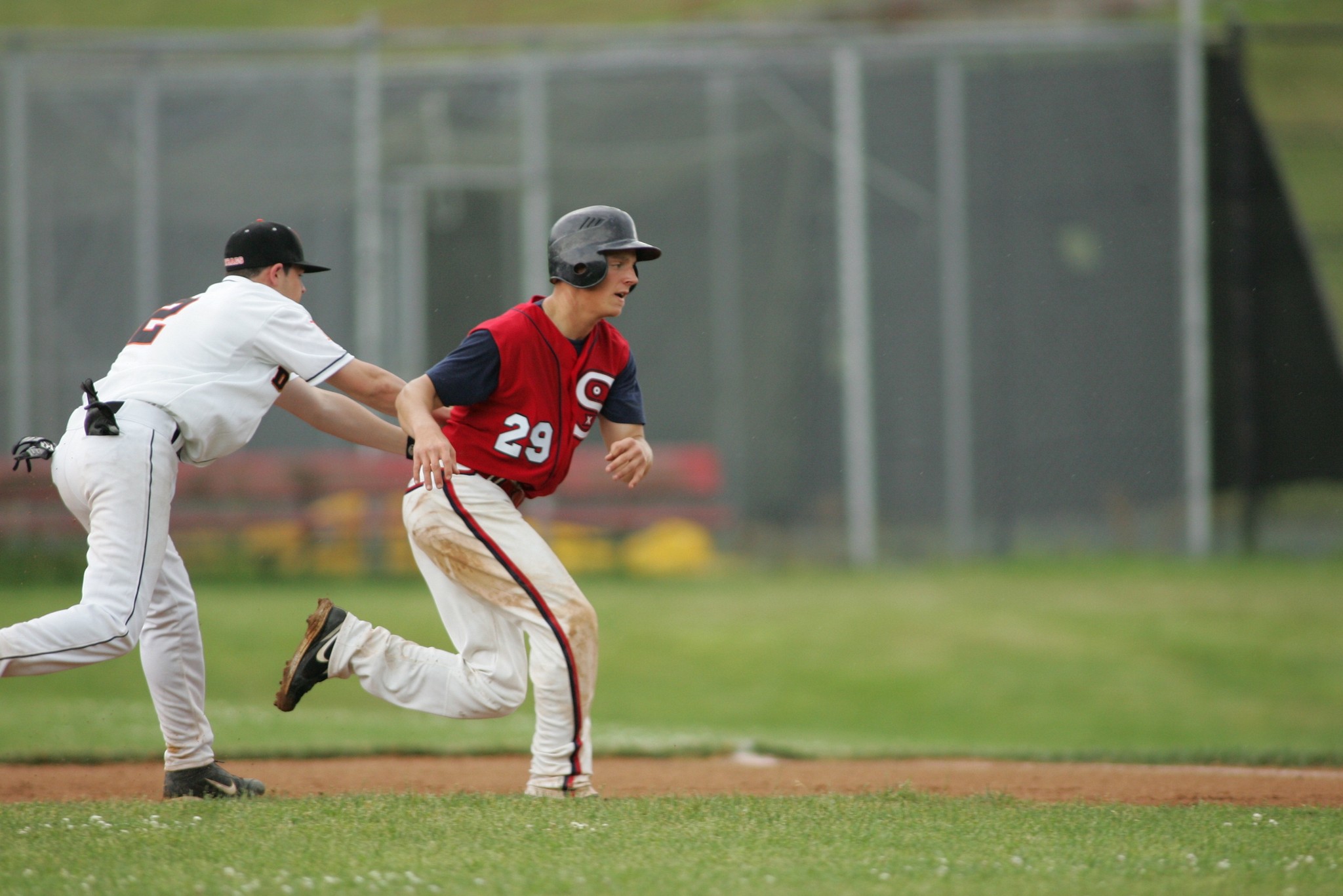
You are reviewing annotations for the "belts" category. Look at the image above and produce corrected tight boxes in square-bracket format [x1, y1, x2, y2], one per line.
[97, 400, 183, 454]
[473, 469, 524, 508]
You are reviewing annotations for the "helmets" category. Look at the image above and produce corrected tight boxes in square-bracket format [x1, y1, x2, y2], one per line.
[548, 205, 661, 294]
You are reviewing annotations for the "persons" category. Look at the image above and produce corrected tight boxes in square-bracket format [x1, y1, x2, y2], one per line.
[2, 222, 453, 798]
[271, 204, 661, 796]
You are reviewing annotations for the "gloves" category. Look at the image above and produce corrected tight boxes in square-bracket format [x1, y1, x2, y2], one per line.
[80, 378, 121, 436]
[12, 435, 57, 472]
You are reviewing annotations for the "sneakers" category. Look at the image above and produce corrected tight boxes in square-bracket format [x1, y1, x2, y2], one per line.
[274, 599, 346, 712]
[164, 761, 266, 803]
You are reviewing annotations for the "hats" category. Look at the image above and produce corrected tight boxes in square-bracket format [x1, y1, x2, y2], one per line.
[224, 218, 331, 273]
